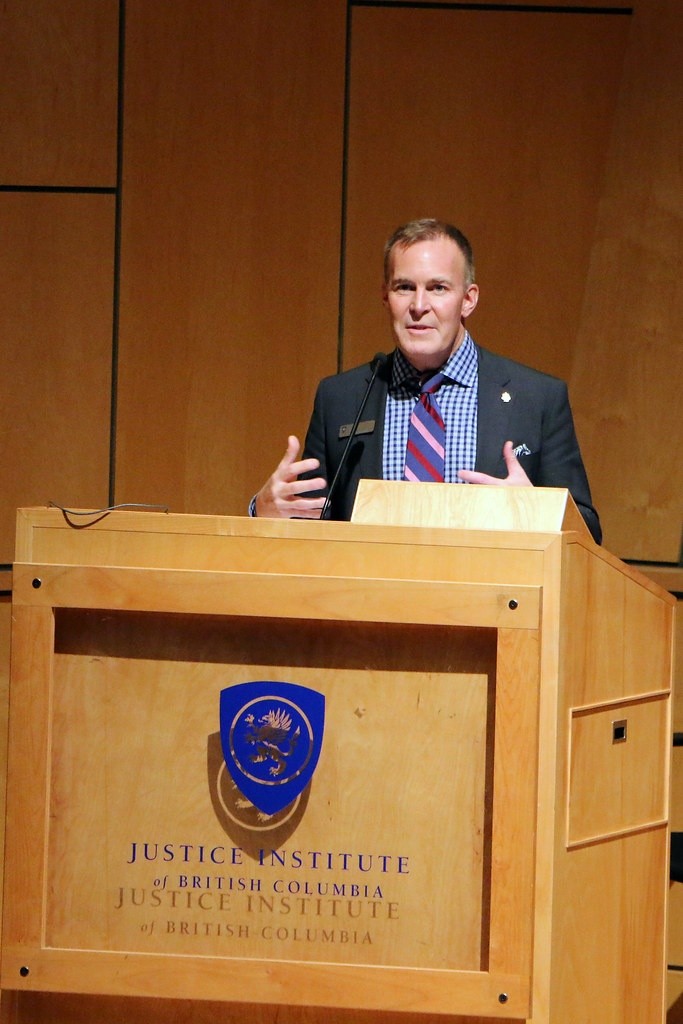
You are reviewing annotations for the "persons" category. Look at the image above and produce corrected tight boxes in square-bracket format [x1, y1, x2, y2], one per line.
[248, 217, 602, 546]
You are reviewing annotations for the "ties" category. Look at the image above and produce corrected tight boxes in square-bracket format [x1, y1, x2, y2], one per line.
[401, 371, 449, 482]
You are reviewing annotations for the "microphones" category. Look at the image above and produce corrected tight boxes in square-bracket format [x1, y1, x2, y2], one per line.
[318, 352, 387, 521]
